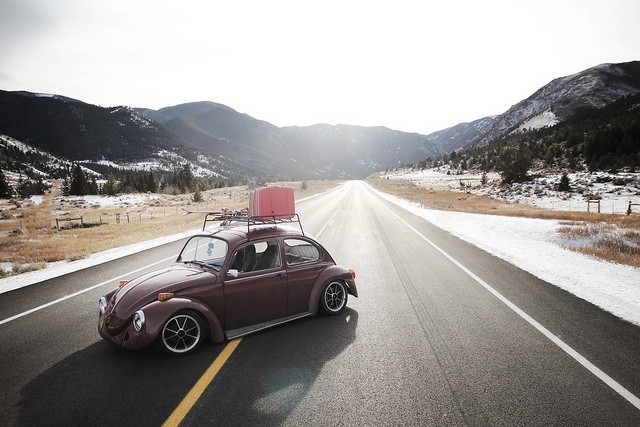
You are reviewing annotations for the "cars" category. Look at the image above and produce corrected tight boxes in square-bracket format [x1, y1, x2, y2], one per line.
[97, 221, 358, 356]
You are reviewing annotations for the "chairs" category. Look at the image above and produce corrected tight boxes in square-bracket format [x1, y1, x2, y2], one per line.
[230, 245, 257, 272]
[250, 244, 279, 271]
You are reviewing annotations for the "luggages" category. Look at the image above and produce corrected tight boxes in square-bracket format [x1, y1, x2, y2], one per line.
[249, 183, 295, 221]
